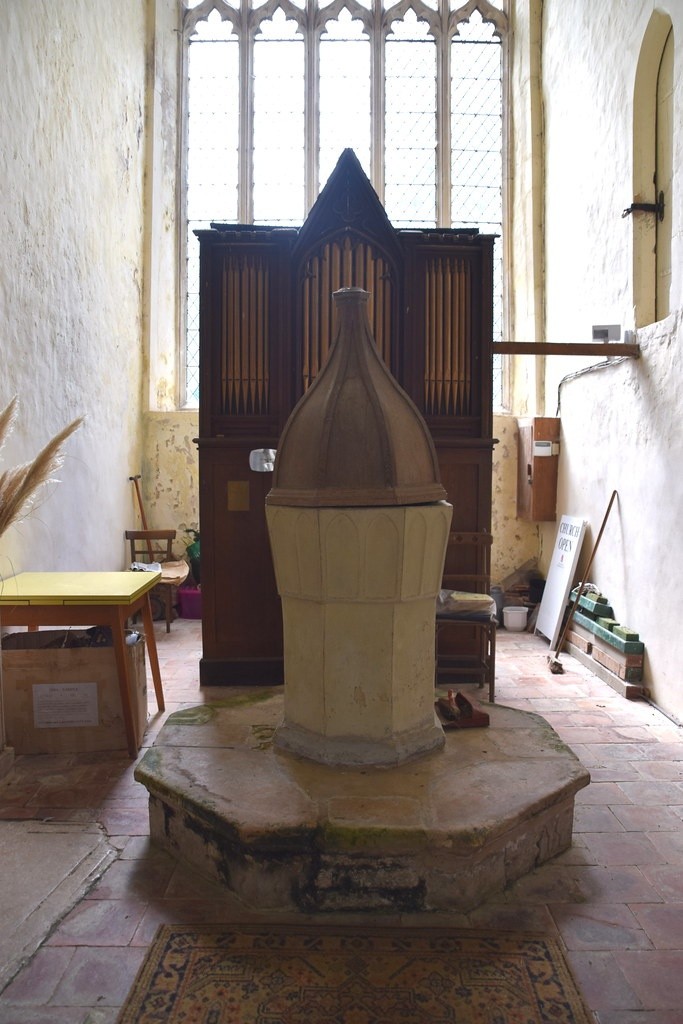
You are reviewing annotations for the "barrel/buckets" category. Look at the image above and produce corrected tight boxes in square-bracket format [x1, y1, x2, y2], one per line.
[503, 606, 529, 632]
[529, 580, 545, 603]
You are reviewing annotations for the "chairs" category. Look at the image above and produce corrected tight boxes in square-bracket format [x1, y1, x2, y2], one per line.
[432, 530, 498, 704]
[123, 529, 178, 634]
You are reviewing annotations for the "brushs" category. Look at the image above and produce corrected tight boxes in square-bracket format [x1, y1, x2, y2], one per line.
[438, 688, 459, 720]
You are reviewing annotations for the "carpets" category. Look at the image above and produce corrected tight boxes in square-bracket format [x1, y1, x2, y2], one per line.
[109, 918, 599, 1024]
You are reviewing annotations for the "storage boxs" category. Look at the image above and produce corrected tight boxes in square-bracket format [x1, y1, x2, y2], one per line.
[0, 628, 150, 756]
[176, 578, 202, 620]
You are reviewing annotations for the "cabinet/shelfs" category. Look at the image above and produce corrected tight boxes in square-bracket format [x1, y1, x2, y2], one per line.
[514, 416, 560, 525]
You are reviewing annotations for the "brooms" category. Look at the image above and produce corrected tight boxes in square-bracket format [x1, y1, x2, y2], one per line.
[547, 489, 617, 674]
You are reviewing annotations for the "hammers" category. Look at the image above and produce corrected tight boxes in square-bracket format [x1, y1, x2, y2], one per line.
[546, 488, 618, 673]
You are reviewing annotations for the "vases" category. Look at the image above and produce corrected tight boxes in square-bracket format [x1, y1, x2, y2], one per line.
[501, 605, 530, 632]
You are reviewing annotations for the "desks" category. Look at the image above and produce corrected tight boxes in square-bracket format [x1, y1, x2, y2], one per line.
[0, 568, 167, 762]
